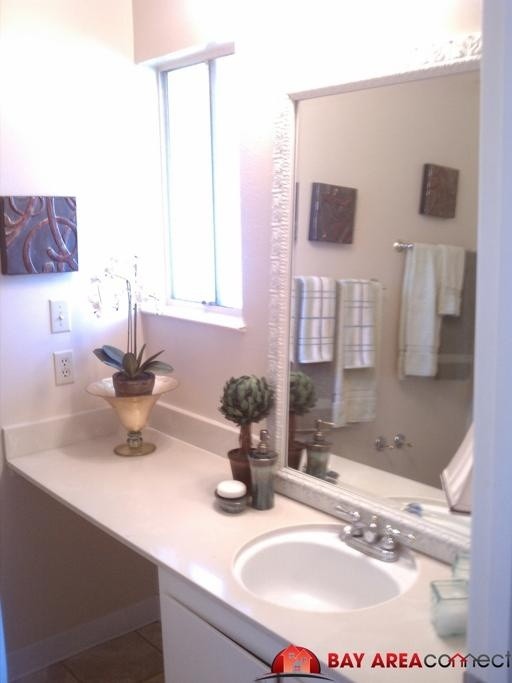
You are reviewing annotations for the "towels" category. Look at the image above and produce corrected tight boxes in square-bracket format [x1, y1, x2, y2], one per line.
[395, 241, 477, 382]
[291, 275, 386, 428]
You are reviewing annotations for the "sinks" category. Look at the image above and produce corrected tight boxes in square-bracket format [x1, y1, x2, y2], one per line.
[233, 522, 421, 614]
[378, 497, 471, 537]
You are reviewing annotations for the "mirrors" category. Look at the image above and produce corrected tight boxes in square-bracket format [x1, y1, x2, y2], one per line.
[265, 33, 486, 572]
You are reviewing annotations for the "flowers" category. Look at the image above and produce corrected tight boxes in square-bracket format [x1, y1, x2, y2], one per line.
[85, 246, 174, 375]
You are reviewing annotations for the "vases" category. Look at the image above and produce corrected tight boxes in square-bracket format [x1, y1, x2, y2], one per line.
[112, 372, 155, 396]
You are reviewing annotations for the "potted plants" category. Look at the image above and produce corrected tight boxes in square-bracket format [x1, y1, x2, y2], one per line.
[288, 371, 320, 468]
[217, 373, 276, 495]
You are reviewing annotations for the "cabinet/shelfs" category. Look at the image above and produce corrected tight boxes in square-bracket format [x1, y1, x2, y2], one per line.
[156, 568, 347, 681]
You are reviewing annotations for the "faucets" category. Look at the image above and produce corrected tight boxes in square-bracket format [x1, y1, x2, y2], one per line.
[338, 513, 379, 546]
